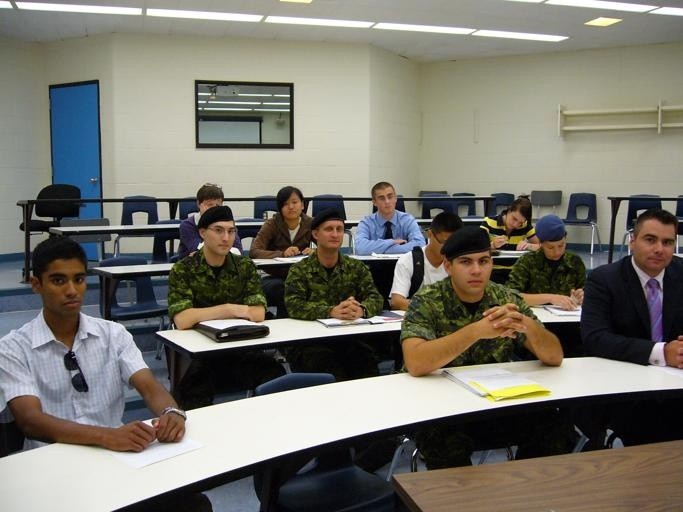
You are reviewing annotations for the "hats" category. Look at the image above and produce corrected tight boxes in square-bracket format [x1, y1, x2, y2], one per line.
[311, 208, 344, 227]
[197, 204, 233, 228]
[440, 225, 491, 260]
[536, 214, 568, 242]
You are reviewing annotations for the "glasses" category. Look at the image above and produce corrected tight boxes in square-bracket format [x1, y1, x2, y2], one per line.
[63, 348, 88, 394]
[431, 230, 445, 247]
[209, 226, 237, 235]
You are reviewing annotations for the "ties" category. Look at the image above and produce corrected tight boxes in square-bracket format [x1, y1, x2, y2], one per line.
[646, 279, 664, 341]
[382, 221, 393, 239]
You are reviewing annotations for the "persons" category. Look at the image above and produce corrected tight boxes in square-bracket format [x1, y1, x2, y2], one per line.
[166, 205, 286, 407]
[352, 181, 426, 257]
[386, 211, 463, 312]
[505, 214, 587, 310]
[0, 236, 212, 512]
[175, 183, 244, 261]
[283, 207, 386, 378]
[397, 226, 564, 469]
[477, 193, 542, 256]
[573, 208, 682, 446]
[247, 186, 313, 306]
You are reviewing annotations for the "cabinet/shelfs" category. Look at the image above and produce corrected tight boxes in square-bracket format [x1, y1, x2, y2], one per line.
[557, 99, 683, 138]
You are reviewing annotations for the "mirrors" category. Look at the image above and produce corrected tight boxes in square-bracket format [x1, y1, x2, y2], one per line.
[195, 80, 293, 148]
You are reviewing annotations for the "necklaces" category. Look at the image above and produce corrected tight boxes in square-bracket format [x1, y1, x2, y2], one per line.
[285, 221, 300, 230]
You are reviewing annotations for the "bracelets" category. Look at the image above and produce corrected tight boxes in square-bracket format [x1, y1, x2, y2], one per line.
[161, 406, 186, 418]
[492, 242, 495, 248]
[358, 304, 366, 318]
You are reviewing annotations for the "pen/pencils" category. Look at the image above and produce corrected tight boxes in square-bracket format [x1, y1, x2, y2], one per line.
[382, 318, 401, 320]
[492, 235, 509, 244]
